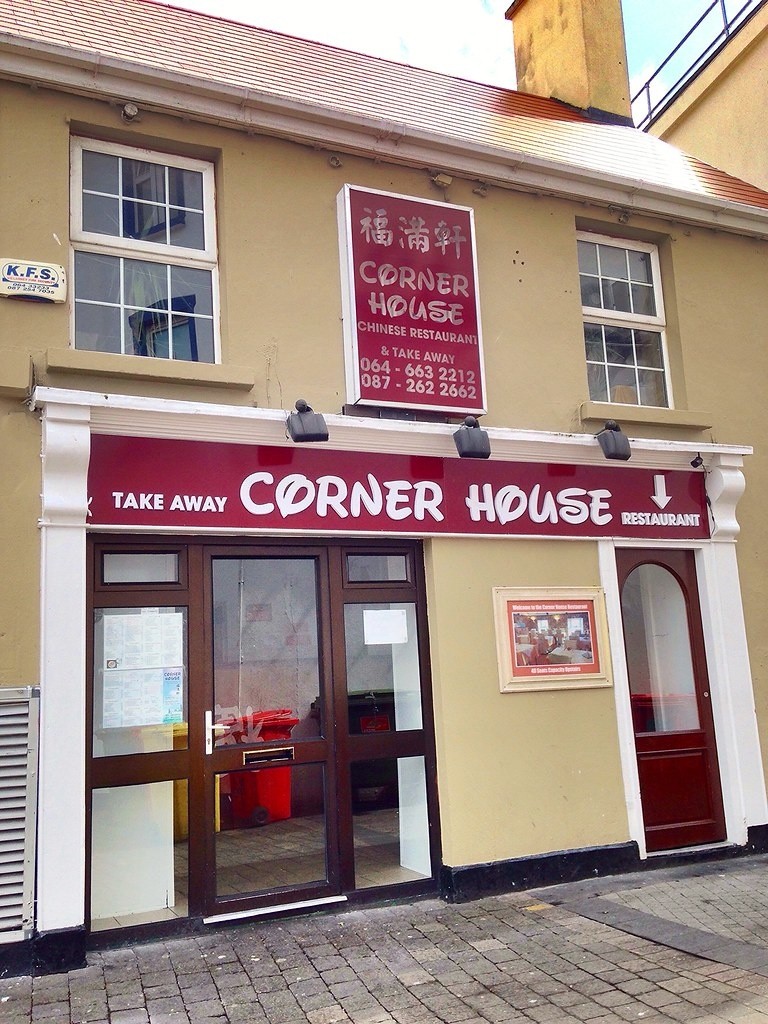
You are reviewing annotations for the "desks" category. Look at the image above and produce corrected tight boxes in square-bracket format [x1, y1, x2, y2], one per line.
[517, 635, 555, 648]
[565, 637, 590, 649]
[548, 648, 592, 663]
[516, 643, 535, 657]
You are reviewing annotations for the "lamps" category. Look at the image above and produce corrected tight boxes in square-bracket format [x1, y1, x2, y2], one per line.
[596, 421, 631, 461]
[433, 173, 452, 188]
[121, 104, 138, 125]
[286, 400, 329, 442]
[453, 416, 490, 459]
[691, 457, 703, 468]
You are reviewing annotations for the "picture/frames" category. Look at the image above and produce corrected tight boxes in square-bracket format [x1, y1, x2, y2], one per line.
[492, 586, 614, 693]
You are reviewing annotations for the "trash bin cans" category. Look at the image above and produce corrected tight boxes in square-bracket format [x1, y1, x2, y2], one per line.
[224, 707, 300, 827]
[632, 692, 696, 732]
[310, 687, 400, 813]
[138, 722, 231, 842]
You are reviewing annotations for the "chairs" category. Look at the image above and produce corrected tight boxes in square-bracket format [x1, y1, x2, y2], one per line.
[517, 647, 540, 666]
